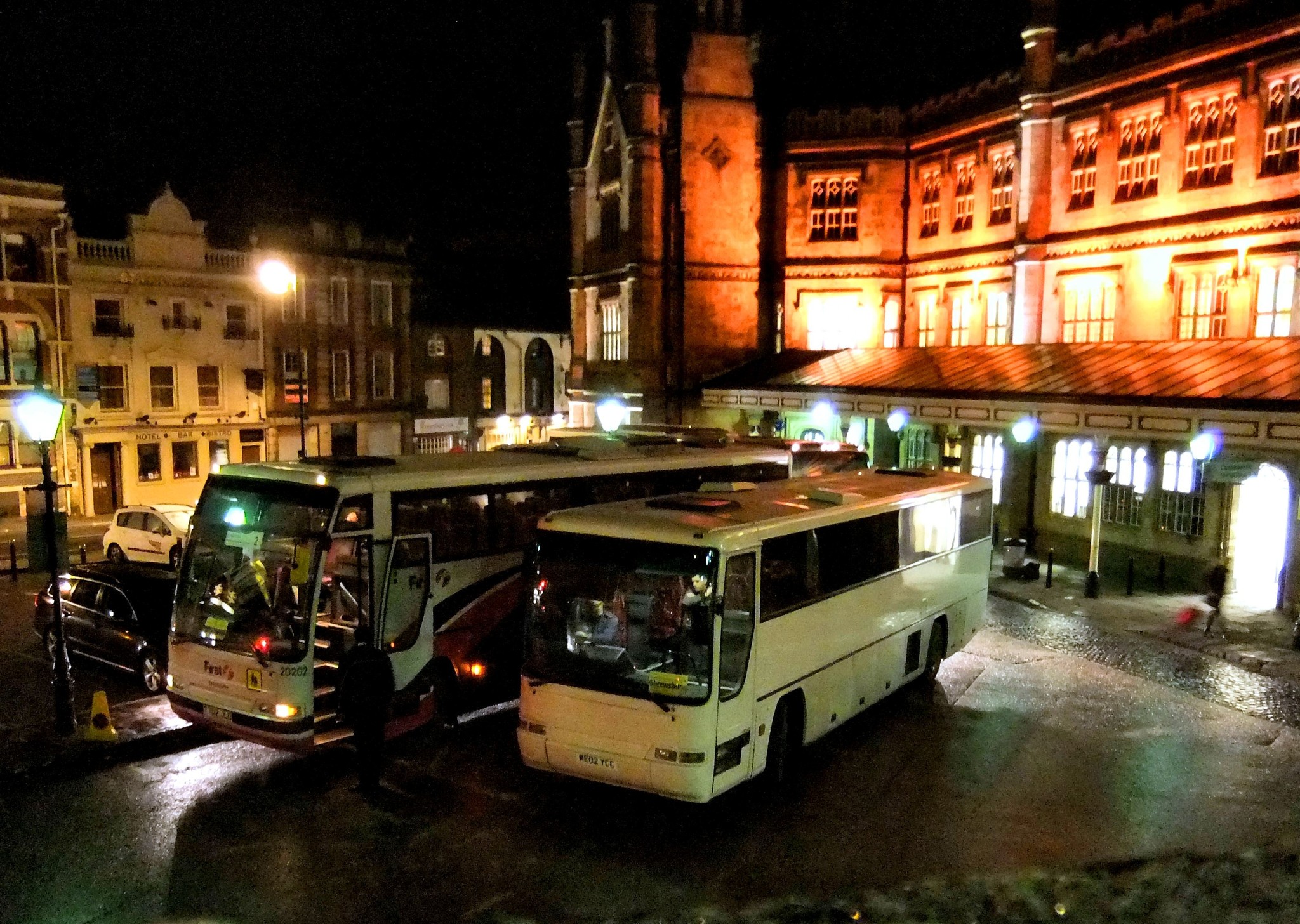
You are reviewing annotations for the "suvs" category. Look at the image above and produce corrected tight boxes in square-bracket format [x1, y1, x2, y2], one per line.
[31, 562, 181, 695]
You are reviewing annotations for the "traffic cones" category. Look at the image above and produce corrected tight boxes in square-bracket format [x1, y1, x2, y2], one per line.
[83, 690, 117, 741]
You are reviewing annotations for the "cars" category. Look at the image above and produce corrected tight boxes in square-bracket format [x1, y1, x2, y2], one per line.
[102, 504, 197, 570]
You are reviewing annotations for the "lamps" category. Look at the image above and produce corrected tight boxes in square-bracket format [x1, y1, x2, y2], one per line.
[136, 415, 149, 422]
[187, 413, 198, 419]
[236, 411, 246, 417]
[85, 417, 94, 423]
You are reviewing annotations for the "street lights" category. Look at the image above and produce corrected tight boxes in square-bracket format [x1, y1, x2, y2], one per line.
[260, 260, 307, 461]
[12, 387, 78, 736]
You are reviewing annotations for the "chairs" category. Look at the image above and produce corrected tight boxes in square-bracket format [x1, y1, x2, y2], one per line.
[397, 497, 540, 555]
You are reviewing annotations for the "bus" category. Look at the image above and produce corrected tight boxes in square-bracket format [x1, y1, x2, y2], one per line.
[515, 465, 992, 804]
[165, 423, 793, 760]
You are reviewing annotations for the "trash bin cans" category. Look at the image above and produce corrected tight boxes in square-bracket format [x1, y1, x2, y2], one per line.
[1002, 538, 1027, 578]
[25, 511, 70, 574]
[1084, 571, 1098, 598]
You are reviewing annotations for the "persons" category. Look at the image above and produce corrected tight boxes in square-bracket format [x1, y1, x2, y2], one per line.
[668, 570, 714, 673]
[1202, 558, 1230, 638]
[572, 598, 622, 649]
